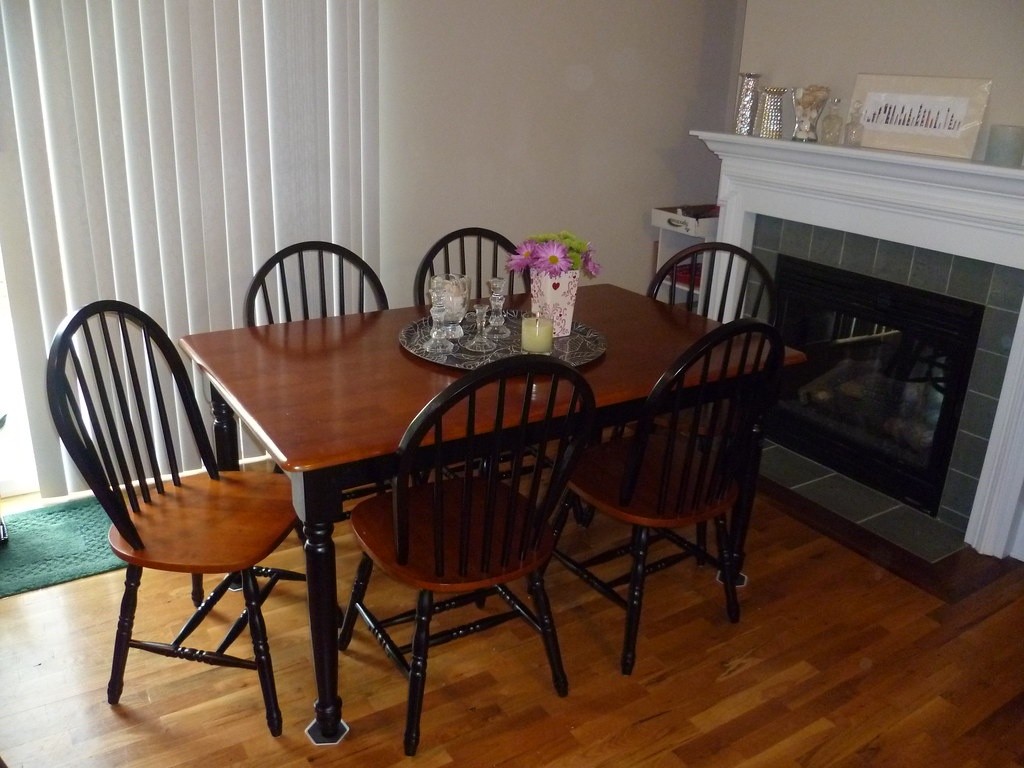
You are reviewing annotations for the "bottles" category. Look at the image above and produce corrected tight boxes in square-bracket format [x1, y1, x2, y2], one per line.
[844, 99, 865, 146]
[821, 98, 844, 146]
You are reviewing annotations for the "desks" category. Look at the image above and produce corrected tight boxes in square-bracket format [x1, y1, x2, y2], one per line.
[178, 283, 807, 739]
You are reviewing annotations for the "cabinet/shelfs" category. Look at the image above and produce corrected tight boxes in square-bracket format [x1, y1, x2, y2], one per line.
[650, 203, 720, 316]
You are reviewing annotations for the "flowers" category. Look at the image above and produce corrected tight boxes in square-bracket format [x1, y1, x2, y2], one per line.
[505, 229, 603, 279]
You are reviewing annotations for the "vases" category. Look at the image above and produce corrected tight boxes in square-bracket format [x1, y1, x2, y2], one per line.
[529, 267, 580, 339]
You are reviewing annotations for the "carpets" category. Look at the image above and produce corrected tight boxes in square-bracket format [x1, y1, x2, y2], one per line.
[0, 496, 130, 600]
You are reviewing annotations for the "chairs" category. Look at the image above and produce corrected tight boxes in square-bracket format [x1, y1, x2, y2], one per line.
[584, 241, 779, 568]
[242, 227, 584, 529]
[526, 318, 786, 678]
[335, 355, 597, 757]
[45, 300, 346, 737]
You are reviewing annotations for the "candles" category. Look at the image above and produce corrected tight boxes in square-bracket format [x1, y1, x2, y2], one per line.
[522, 317, 552, 353]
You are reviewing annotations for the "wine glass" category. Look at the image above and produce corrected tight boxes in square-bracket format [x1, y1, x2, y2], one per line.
[431, 274, 471, 338]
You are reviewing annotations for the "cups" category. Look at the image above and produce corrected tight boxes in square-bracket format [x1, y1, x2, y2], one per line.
[791, 87, 828, 143]
